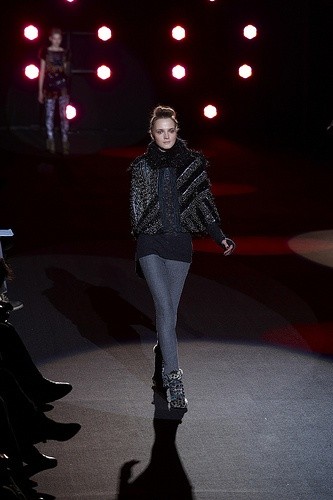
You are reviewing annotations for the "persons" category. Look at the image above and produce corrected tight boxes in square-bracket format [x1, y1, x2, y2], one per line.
[0, 240, 82, 500]
[38, 27, 75, 152]
[130, 106, 236, 408]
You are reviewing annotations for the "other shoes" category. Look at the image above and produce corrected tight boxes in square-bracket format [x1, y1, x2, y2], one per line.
[162, 368, 188, 409]
[36, 377, 73, 403]
[24, 444, 57, 470]
[29, 418, 81, 444]
[153, 345, 165, 395]
